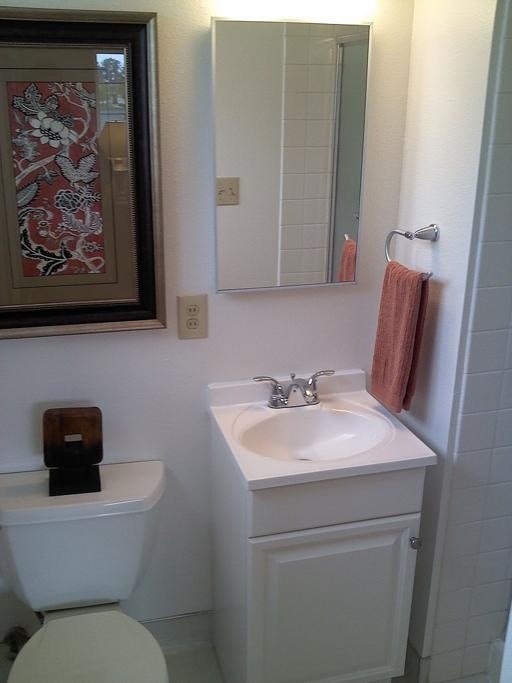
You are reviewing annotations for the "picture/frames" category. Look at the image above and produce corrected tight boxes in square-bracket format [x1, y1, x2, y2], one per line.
[1, 7, 168, 341]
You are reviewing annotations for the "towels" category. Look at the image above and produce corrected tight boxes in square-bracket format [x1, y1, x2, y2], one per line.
[339, 237, 355, 283]
[371, 262, 430, 413]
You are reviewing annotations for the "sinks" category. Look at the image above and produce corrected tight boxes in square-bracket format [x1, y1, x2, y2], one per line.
[232, 400, 396, 462]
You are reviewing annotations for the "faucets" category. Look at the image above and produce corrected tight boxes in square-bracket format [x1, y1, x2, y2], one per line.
[285, 379, 317, 409]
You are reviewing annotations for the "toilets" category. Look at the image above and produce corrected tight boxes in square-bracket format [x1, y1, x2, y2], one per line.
[0, 460, 171, 683]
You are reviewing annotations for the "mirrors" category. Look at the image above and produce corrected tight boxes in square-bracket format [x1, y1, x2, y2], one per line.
[210, 15, 369, 294]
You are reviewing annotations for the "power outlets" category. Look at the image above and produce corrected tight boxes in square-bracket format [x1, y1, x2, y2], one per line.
[216, 176, 239, 205]
[174, 291, 209, 340]
